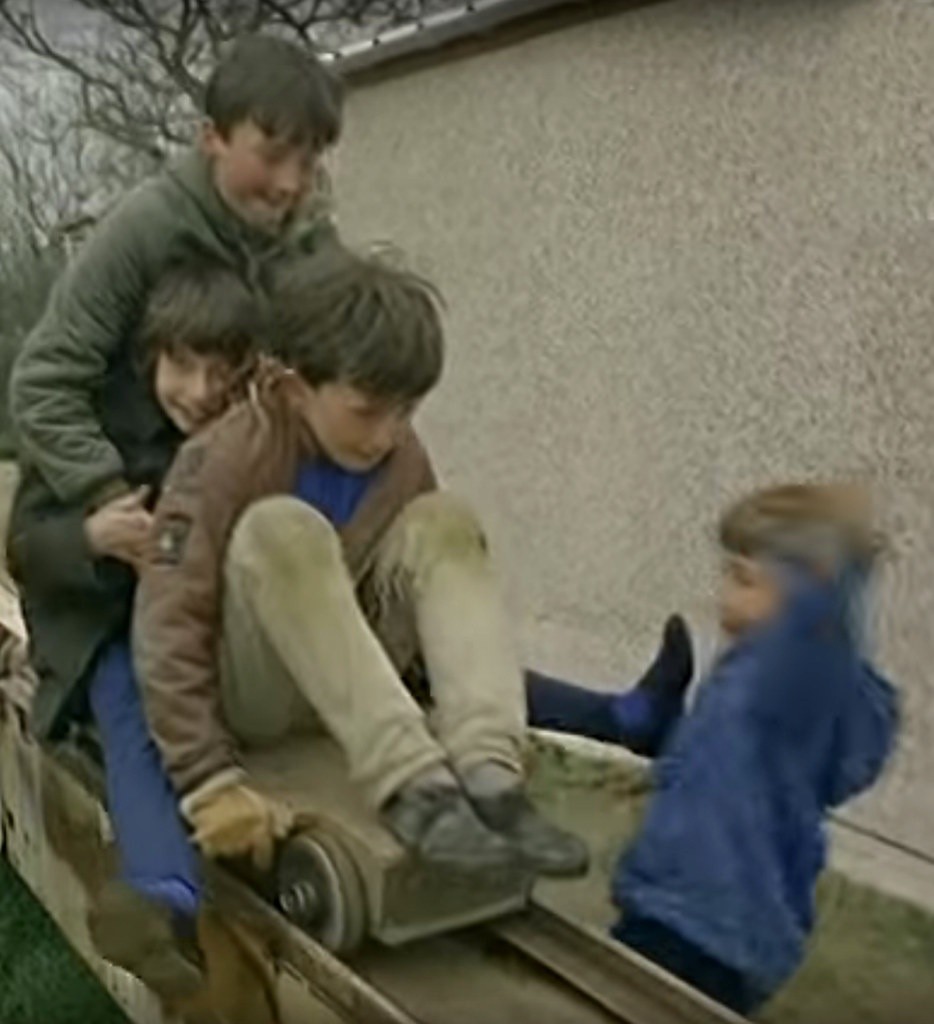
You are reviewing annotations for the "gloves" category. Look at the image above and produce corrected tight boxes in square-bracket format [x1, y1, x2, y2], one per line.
[194, 785, 286, 876]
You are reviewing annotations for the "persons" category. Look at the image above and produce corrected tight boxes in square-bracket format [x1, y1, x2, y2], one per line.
[1, 33, 692, 1004]
[607, 483, 900, 1013]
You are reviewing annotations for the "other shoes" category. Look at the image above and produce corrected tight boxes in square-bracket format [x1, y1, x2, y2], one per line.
[471, 782, 590, 879]
[379, 785, 523, 886]
[87, 881, 205, 1000]
[633, 614, 693, 757]
